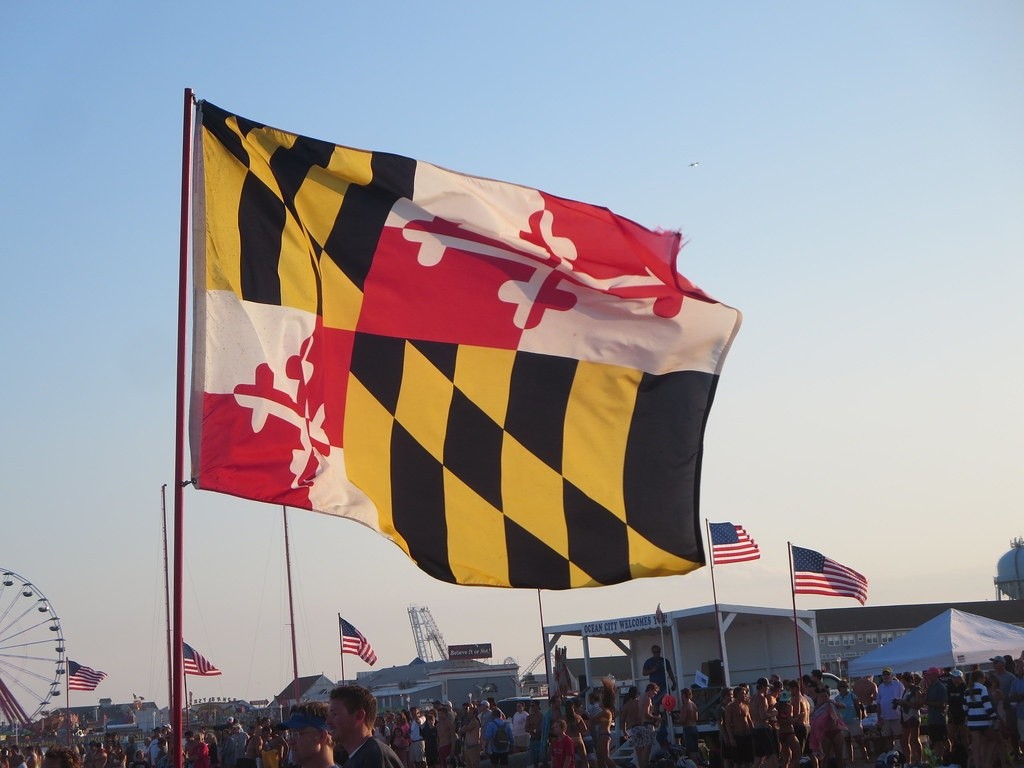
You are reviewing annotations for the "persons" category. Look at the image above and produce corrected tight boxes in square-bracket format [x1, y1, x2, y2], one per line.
[0, 645, 1024, 768]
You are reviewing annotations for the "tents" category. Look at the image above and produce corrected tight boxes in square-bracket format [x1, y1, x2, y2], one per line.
[848, 608, 1024, 694]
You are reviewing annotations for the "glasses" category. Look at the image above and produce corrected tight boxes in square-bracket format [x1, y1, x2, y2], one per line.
[284, 731, 321, 740]
[654, 651, 660, 653]
[194, 738, 200, 740]
[884, 674, 889, 676]
[993, 662, 998, 664]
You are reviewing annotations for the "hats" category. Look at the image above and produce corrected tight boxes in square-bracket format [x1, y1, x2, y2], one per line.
[432, 700, 441, 704]
[951, 670, 963, 677]
[275, 712, 333, 733]
[194, 733, 200, 738]
[924, 667, 939, 673]
[152, 727, 161, 731]
[989, 656, 1005, 662]
[479, 700, 490, 707]
[164, 724, 172, 730]
[779, 691, 792, 702]
[882, 667, 892, 674]
[757, 677, 773, 687]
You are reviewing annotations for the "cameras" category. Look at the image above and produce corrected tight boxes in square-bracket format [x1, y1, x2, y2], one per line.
[892, 699, 899, 710]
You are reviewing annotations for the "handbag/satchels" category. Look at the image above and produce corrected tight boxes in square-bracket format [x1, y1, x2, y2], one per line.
[854, 700, 868, 720]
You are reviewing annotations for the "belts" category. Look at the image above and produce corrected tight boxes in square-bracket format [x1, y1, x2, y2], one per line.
[411, 739, 425, 742]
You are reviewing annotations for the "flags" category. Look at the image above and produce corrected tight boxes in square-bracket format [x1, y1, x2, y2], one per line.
[182, 641, 222, 677]
[792, 545, 868, 607]
[69, 660, 108, 691]
[709, 522, 760, 565]
[339, 616, 378, 667]
[188, 96, 742, 591]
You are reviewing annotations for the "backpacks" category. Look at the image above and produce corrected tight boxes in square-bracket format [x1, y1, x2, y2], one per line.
[491, 720, 510, 750]
[410, 715, 432, 737]
